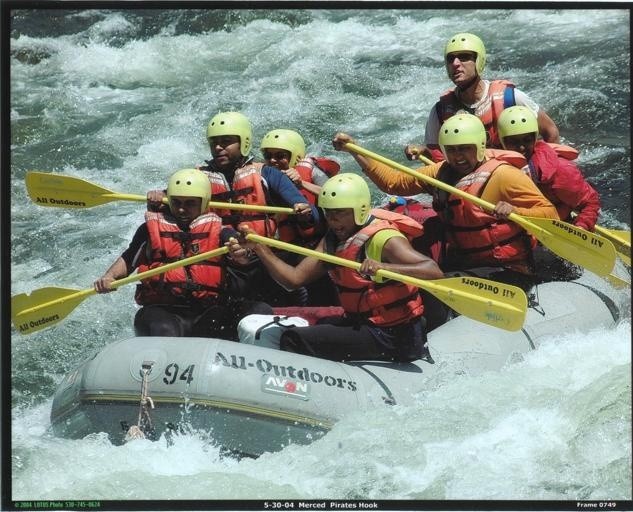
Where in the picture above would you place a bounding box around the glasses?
[447,53,475,64]
[263,151,288,161]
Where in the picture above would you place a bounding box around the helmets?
[167,169,211,216]
[497,105,539,151]
[207,112,254,158]
[439,114,487,163]
[317,173,372,226]
[444,33,486,76]
[259,129,306,169]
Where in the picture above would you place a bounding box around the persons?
[404,106,601,230]
[240,173,442,363]
[259,126,326,207]
[332,113,560,325]
[406,33,559,144]
[95,169,248,335]
[147,113,321,300]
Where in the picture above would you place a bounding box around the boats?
[49,255,631,459]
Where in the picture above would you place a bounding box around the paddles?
[26,172,300,214]
[11,237,242,336]
[237,225,528,332]
[339,142,617,279]
[411,148,633,270]
